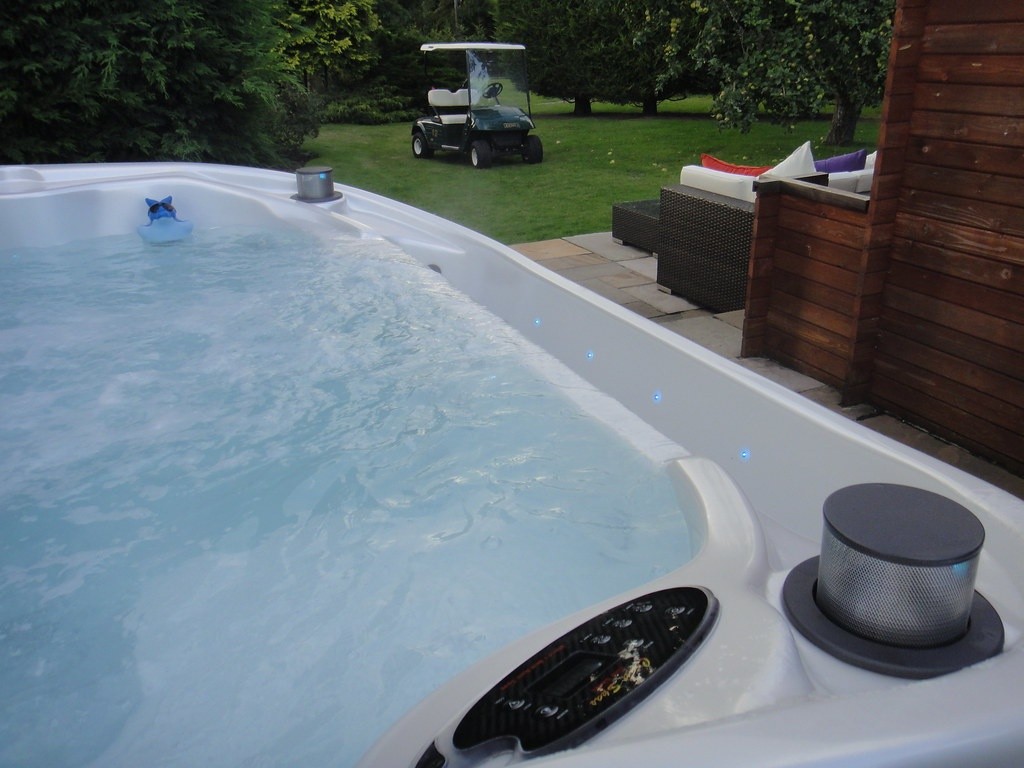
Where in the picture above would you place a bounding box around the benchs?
[428,89,480,124]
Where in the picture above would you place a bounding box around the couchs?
[656,141,877,314]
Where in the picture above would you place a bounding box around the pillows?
[814,148,867,173]
[701,153,772,177]
[757,141,817,177]
